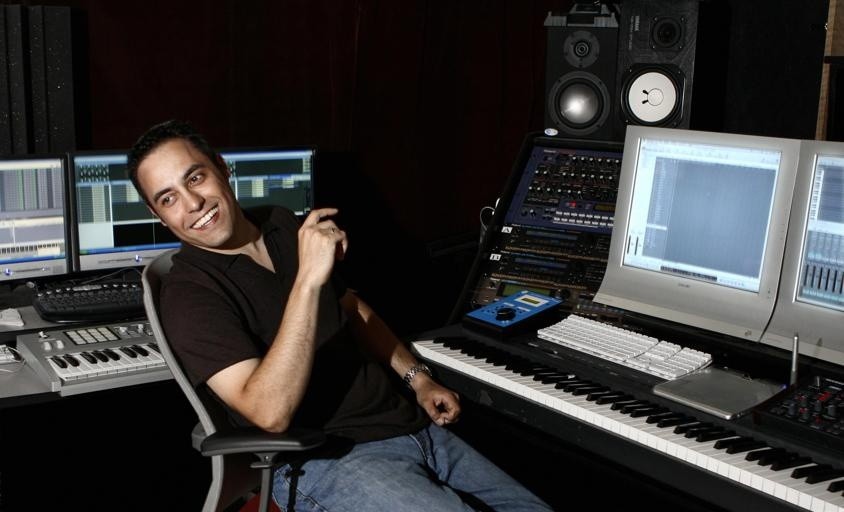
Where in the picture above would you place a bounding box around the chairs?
[142,247,324,511]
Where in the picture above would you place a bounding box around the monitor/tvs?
[758,140,844,366]
[66,148,181,271]
[592,123,802,341]
[216,143,317,218]
[0,154,72,282]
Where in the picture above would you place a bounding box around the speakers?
[611,0,699,141]
[542,0,619,141]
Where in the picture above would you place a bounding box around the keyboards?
[537,314,713,381]
[31,281,146,323]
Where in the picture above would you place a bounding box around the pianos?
[17,321,175,397]
[412,332,840,512]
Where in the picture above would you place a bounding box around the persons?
[127,120,551,512]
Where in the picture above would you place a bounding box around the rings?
[329,226,334,233]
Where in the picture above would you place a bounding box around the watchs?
[405,363,434,385]
